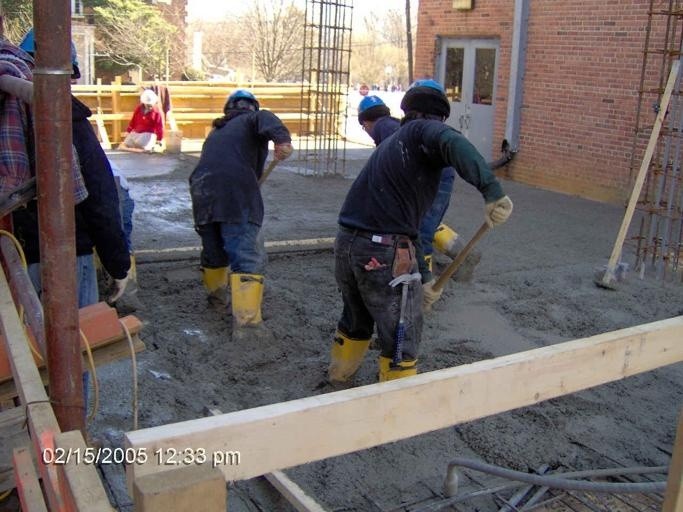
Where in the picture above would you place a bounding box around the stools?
[339,224,375,241]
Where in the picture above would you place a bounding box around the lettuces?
[388,272,422,363]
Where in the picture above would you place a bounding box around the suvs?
[165,130,183,155]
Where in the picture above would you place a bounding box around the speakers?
[199,267,230,293]
[231,273,270,338]
[425,223,481,278]
[327,330,371,382]
[376,356,417,382]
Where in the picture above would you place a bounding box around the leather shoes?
[483,195,512,229]
[422,281,444,308]
[107,268,131,303]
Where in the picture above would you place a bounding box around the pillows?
[140,90,158,105]
[400,78,450,117]
[21,27,81,80]
[224,90,259,110]
[358,96,386,121]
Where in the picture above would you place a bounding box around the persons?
[189,89,294,339]
[324,78,513,382]
[116,89,164,152]
[11,25,130,428]
[358,96,483,284]
